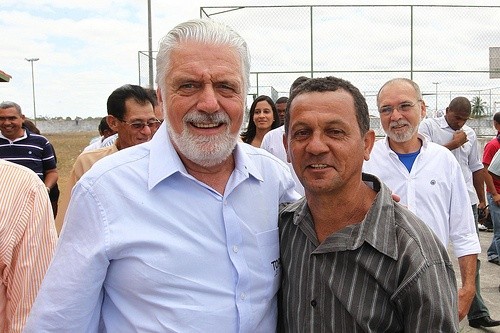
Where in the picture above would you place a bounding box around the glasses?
[378,100,422,115]
[114,114,161,131]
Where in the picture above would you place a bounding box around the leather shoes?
[469,316,500,328]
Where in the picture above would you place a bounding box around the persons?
[3,98,500,328]
[364,79,480,324]
[277,76,462,332]
[0,157,59,333]
[21,19,409,333]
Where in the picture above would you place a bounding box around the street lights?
[24,57,40,127]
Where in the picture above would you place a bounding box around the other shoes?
[490,257,500,266]
[478,224,493,232]
